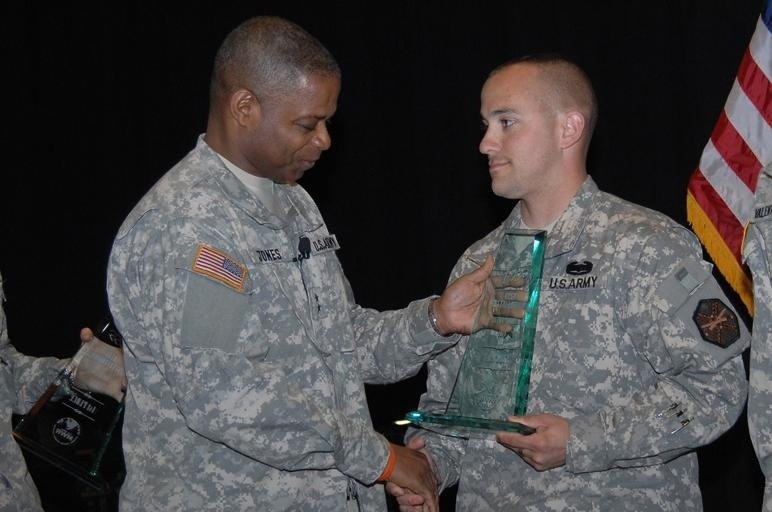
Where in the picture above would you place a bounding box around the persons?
[0,276,129,510]
[748,159,771,511]
[105,14,532,512]
[383,51,750,511]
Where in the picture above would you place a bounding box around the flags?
[686,0,772,319]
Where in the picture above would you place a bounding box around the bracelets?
[429,294,449,338]
[373,441,397,485]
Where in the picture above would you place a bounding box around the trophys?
[7,307,131,499]
[403,227,548,442]
